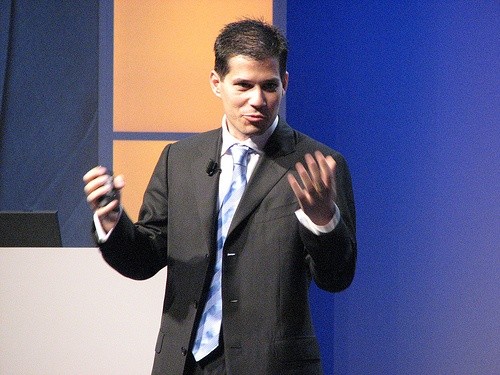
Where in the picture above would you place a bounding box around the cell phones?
[97,165,121,212]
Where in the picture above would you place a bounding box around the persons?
[83,17,359,375]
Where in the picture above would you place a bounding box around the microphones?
[205,158,221,177]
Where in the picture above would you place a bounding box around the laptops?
[0,210,63,247]
